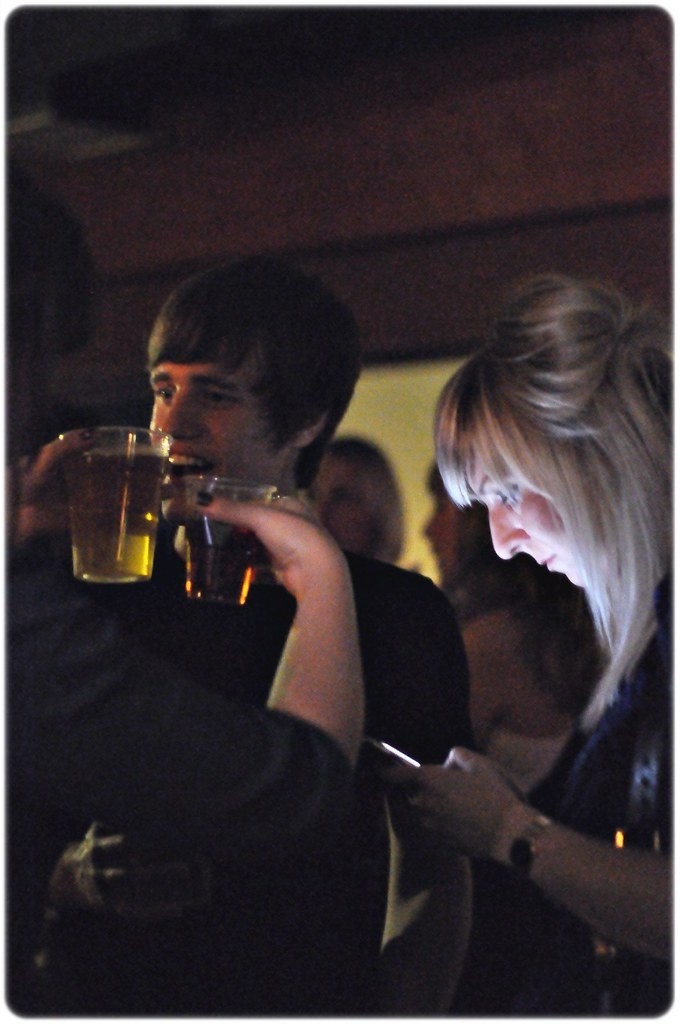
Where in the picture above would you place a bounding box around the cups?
[59,424,173,584]
[187,474,277,605]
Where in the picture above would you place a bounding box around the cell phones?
[361,736,420,769]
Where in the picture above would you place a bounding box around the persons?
[35,253,606,1016]
[383,266,673,1016]
[8,165,365,1016]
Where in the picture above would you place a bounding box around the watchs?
[508,816,554,880]
